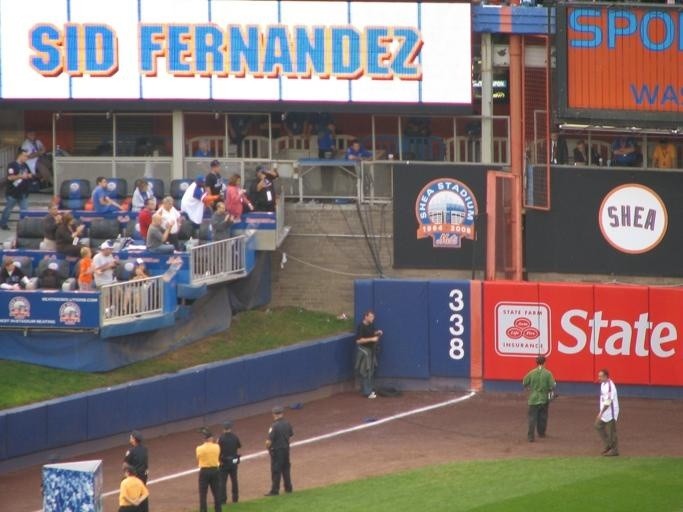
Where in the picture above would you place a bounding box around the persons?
[195,139,215,157]
[218,420,241,505]
[594,368,621,457]
[265,405,295,496]
[225,174,248,219]
[522,355,556,442]
[54,138,157,157]
[230,120,257,158]
[0,172,234,318]
[249,163,279,212]
[122,429,149,485]
[353,309,383,399]
[118,464,150,511]
[343,139,373,194]
[196,431,223,512]
[546,133,679,169]
[205,159,224,210]
[318,117,336,192]
[1,128,53,230]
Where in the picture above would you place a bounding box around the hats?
[210,158,219,165]
[272,406,284,413]
[224,420,232,429]
[197,425,211,438]
[132,430,143,441]
[195,174,207,185]
[99,239,114,250]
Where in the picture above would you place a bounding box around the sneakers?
[600,445,619,456]
[368,391,377,399]
[264,491,279,496]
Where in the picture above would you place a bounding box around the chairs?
[1,176,292,327]
